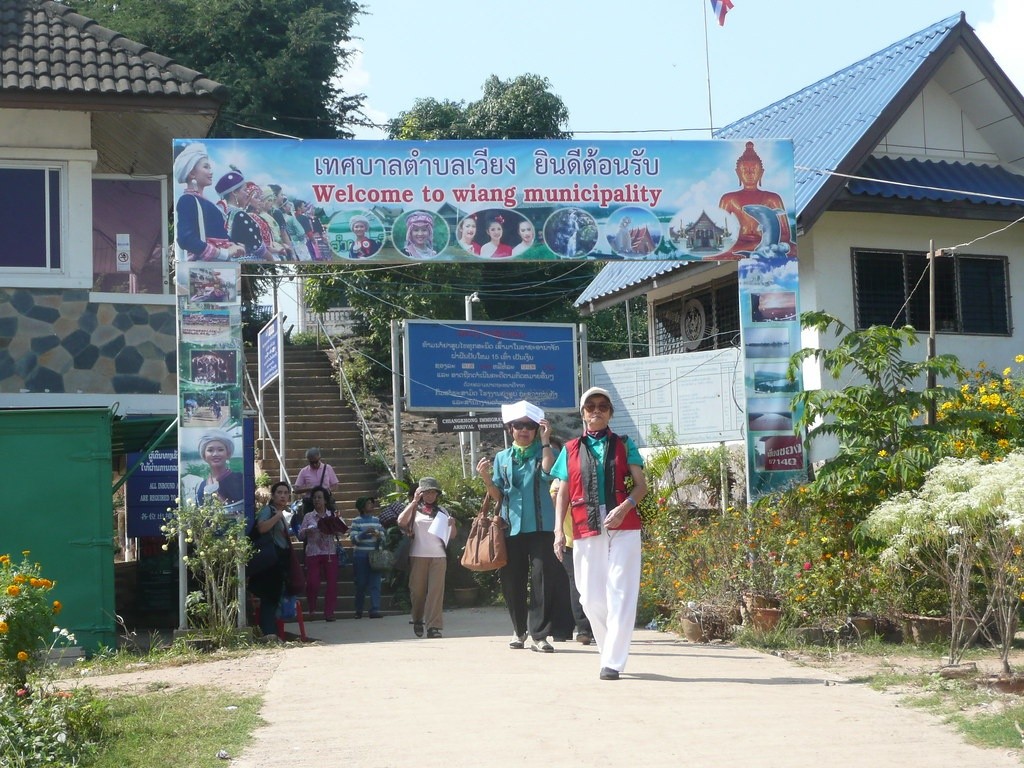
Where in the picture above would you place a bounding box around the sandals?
[509,628,528,650]
[426,627,442,638]
[532,637,554,652]
[414,619,424,637]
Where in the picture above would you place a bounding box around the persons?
[258,183,333,262]
[397,476,457,638]
[540,436,600,646]
[174,142,247,262]
[194,429,245,536]
[511,219,534,256]
[479,211,513,258]
[719,141,799,260]
[401,210,437,258]
[549,387,649,679]
[216,170,276,261]
[475,399,558,653]
[459,212,481,257]
[256,481,290,639]
[348,215,378,259]
[350,496,384,619]
[292,447,340,556]
[244,182,287,262]
[296,485,347,622]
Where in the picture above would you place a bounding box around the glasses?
[309,458,320,465]
[584,403,612,412]
[511,421,538,431]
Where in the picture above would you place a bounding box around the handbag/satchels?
[367,535,389,572]
[334,533,349,567]
[461,486,509,571]
[288,549,304,592]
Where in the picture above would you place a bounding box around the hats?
[356,497,374,514]
[406,212,434,243]
[174,142,312,213]
[580,387,614,419]
[349,216,370,231]
[198,429,234,459]
[418,478,442,495]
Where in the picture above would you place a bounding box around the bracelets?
[627,496,636,507]
[541,443,550,448]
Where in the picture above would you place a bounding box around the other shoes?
[327,614,336,622]
[370,613,383,618]
[576,631,591,645]
[600,666,619,680]
[355,613,361,619]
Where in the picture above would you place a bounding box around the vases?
[851,617,874,638]
[973,676,1024,698]
[680,617,717,643]
[451,587,478,605]
[750,608,784,631]
[896,611,951,645]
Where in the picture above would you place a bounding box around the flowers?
[634,356,1024,676]
